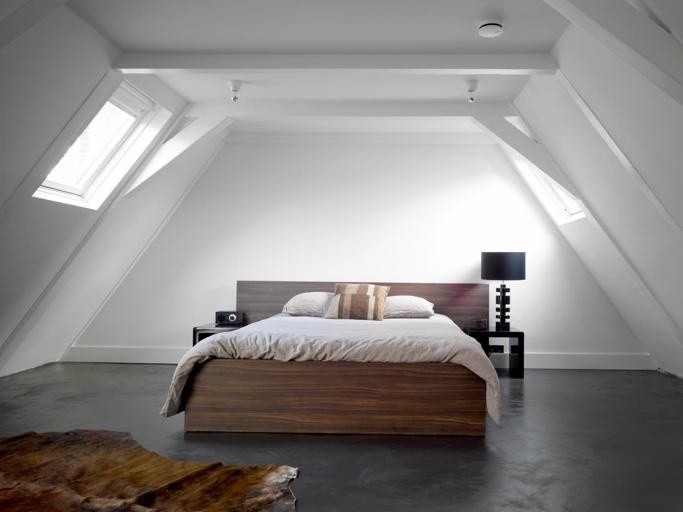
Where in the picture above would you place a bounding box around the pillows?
[281,283,435,322]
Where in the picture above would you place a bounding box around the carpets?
[0,428,301,512]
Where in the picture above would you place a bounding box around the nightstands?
[193,323,239,347]
[464,327,524,379]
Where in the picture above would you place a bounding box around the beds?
[182,280,491,438]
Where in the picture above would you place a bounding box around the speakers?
[214,310,244,326]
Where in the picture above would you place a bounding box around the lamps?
[465,80,477,103]
[481,251,525,332]
[476,20,505,38]
[229,80,241,104]
